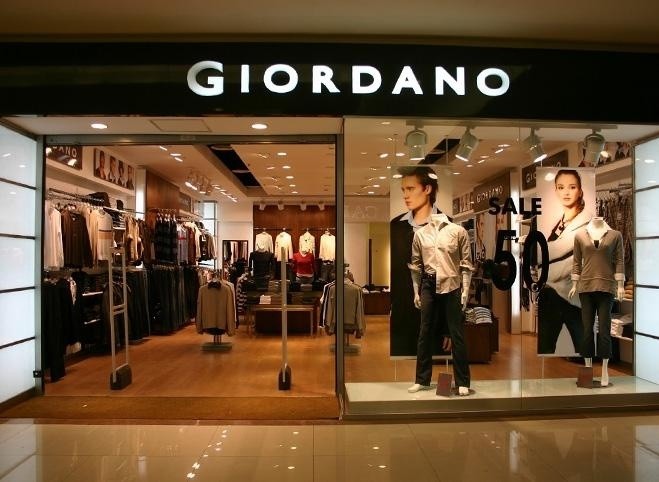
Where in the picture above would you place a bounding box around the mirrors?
[221,239,249,270]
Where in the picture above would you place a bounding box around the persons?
[116,160,125,187]
[105,155,117,183]
[537,170,593,353]
[390,168,452,356]
[567,217,627,386]
[125,166,135,190]
[406,213,472,395]
[95,151,106,180]
[292,247,317,283]
[248,242,276,278]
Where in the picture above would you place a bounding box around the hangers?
[154,206,178,224]
[596,184,634,209]
[149,258,184,273]
[47,189,107,218]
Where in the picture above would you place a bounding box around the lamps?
[184,168,214,197]
[401,118,619,170]
[256,197,328,212]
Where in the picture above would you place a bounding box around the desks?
[247,304,323,338]
